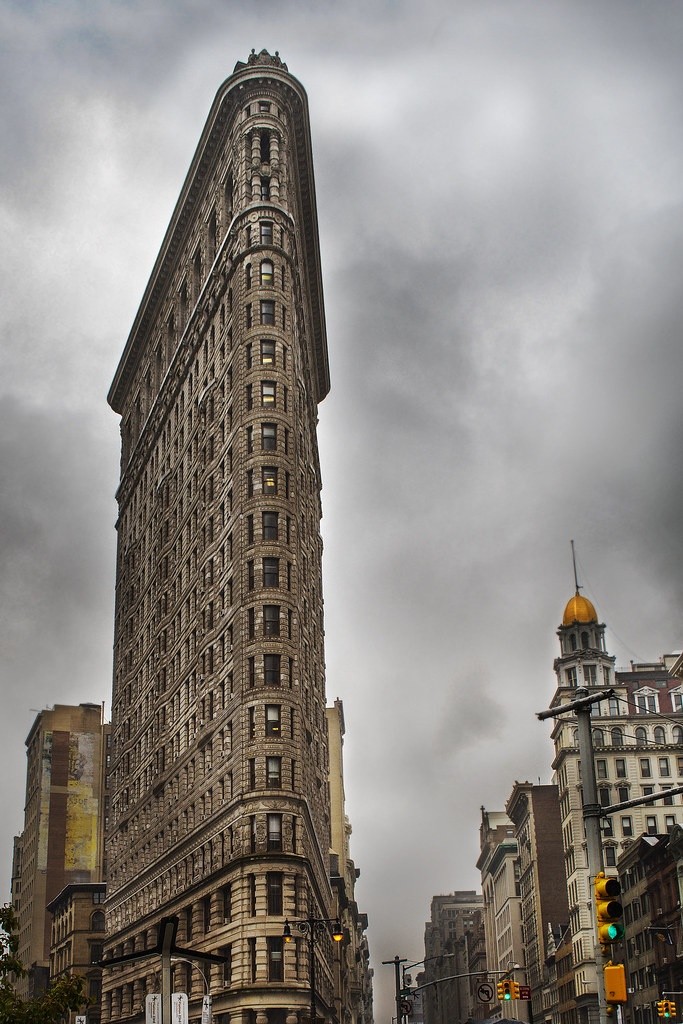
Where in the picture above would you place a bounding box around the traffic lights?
[595,877,625,944]
[497,980,520,1001]
[657,1000,677,1019]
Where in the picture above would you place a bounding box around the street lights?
[170,958,212,1024]
[403,953,455,1024]
[282,904,343,1024]
